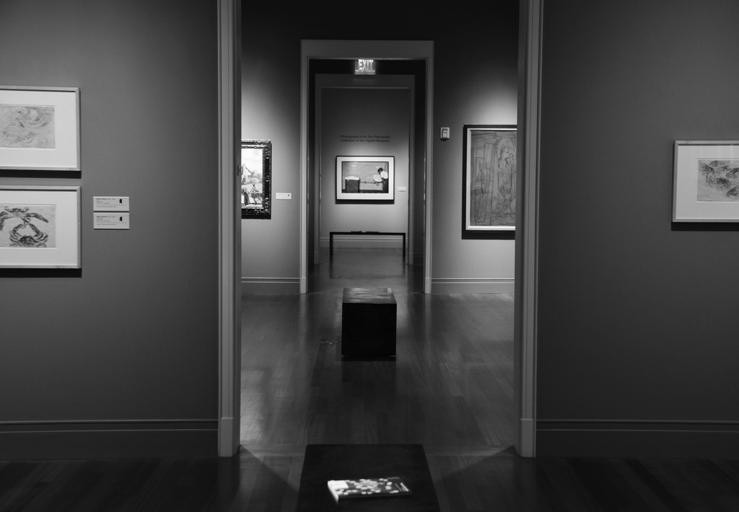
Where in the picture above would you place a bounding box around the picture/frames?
[672,139,739,224]
[240,139,272,219]
[0,84,82,270]
[335,155,395,201]
[462,124,517,240]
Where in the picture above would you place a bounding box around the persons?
[248,184,262,205]
[240,162,250,206]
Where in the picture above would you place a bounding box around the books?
[327,475,414,502]
